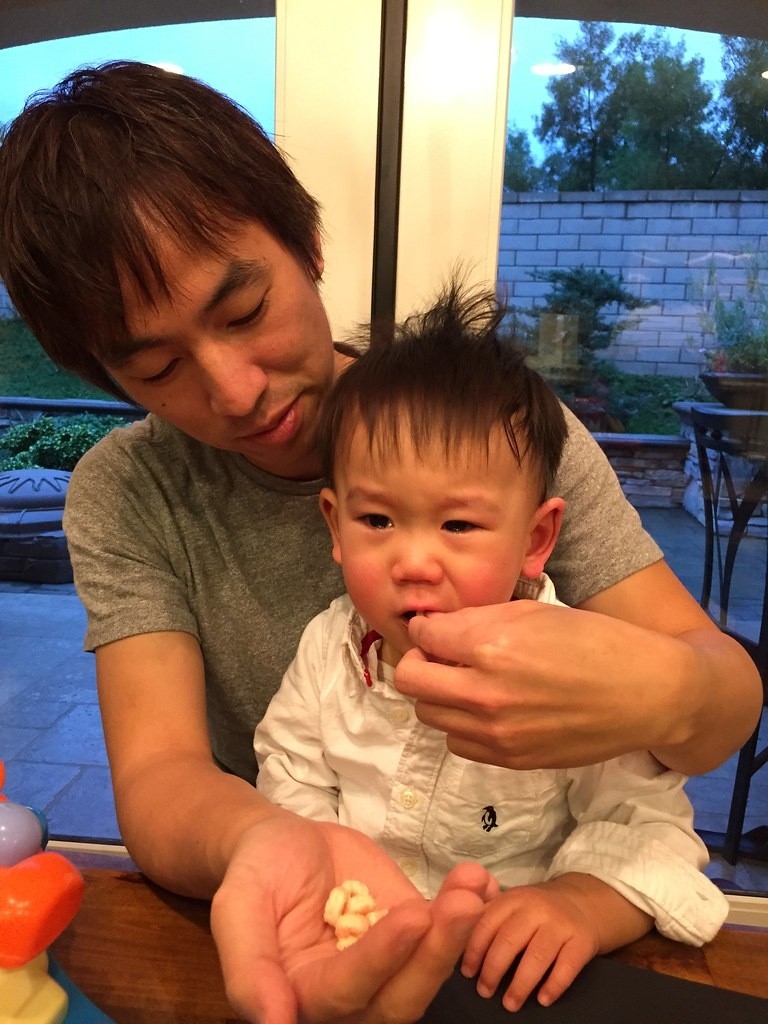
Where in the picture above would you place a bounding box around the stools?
[690,403,768,895]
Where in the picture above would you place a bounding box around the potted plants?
[687,237,768,411]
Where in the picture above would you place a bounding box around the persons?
[249,274,730,1011]
[0,59,763,1024]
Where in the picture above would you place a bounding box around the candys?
[323,879,390,950]
